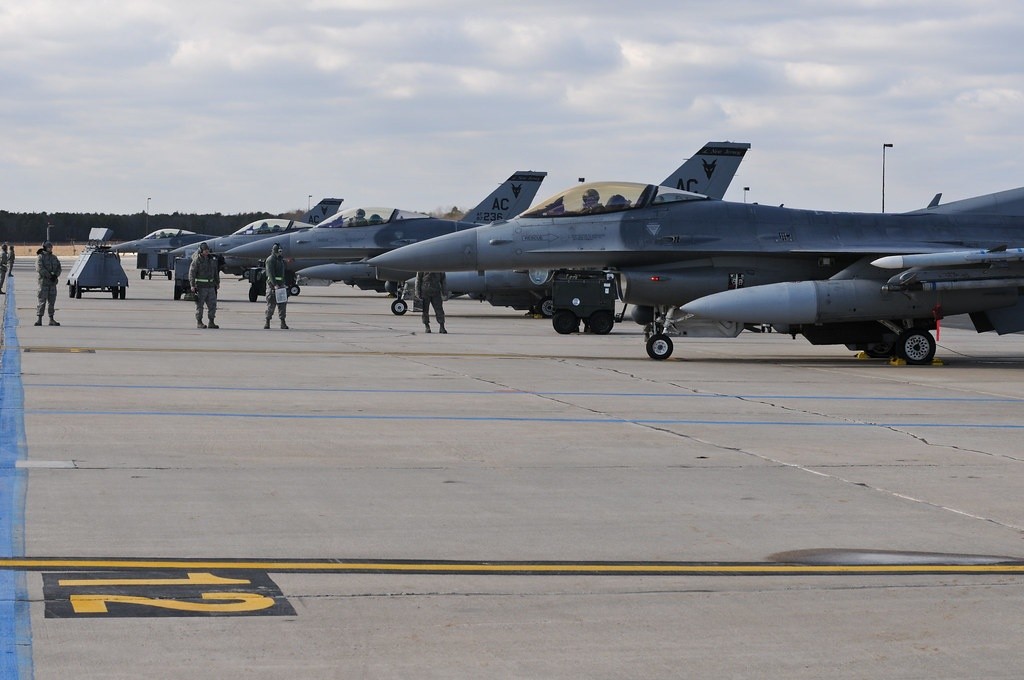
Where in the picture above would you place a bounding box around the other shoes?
[9,273,13,276]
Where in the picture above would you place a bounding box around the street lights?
[881,143,893,213]
[744,186,750,203]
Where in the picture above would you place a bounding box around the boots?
[264,320,270,329]
[440,324,447,333]
[208,319,219,328]
[425,324,431,333]
[197,320,207,328]
[281,321,288,329]
[49,316,60,326]
[0,288,6,294]
[34,316,42,326]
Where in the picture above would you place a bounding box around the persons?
[582,190,605,213]
[350,209,368,227]
[414,272,447,335]
[7,246,15,277]
[188,242,220,328]
[0,245,9,294]
[263,243,290,330]
[33,241,60,326]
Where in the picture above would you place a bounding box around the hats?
[201,243,209,250]
[42,241,52,248]
[272,243,283,253]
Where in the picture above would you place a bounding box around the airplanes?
[365,140,1024,366]
[99,169,660,336]
